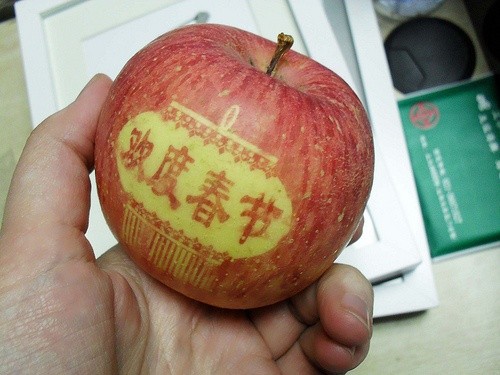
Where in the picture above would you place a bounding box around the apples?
[95,22,376,308]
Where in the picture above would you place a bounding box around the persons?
[0,73,374,375]
